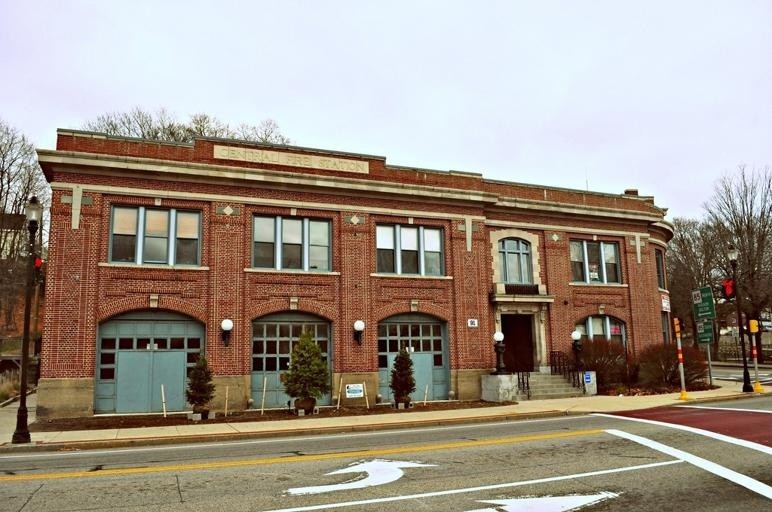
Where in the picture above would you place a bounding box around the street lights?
[11,192,44,444]
[728,243,755,392]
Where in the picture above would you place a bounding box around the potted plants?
[280,325,334,415]
[389,344,417,409]
[185,354,217,420]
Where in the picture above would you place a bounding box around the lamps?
[354,320,365,346]
[222,319,233,346]
